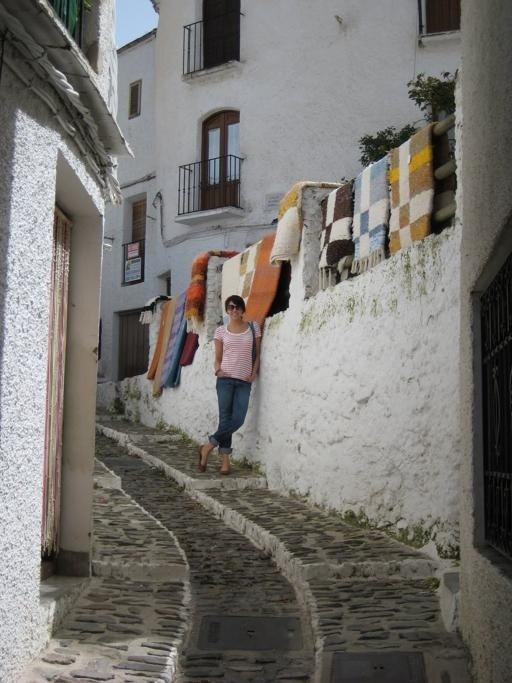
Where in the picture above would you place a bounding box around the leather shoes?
[220,471,229,475]
[199,446,206,472]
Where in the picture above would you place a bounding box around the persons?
[196,294,261,476]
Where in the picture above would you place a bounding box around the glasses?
[228,305,242,310]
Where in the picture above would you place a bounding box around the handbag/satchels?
[248,321,256,362]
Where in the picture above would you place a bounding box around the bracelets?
[215,369,222,376]
